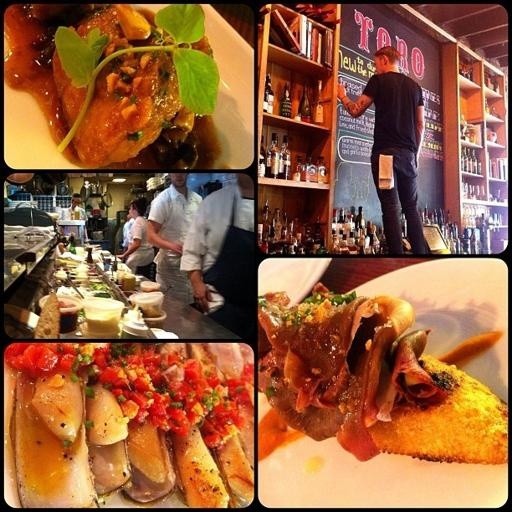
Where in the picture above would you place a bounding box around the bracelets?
[341,95,347,101]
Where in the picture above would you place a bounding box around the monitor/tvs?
[423,224,450,251]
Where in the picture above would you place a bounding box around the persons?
[338,45,432,258]
[114,172,257,339]
[57,193,88,240]
[475,217,485,254]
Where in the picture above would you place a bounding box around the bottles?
[71,211,81,219]
[333,206,509,254]
[263,76,327,256]
[455,63,506,203]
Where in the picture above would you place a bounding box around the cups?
[45,235,166,336]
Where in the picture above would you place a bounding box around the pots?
[11,172,113,208]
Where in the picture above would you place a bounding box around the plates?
[257,255,508,512]
[4,2,253,170]
[259,257,332,323]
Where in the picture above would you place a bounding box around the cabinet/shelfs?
[258,4,508,255]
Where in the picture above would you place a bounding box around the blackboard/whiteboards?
[334,4,445,235]
